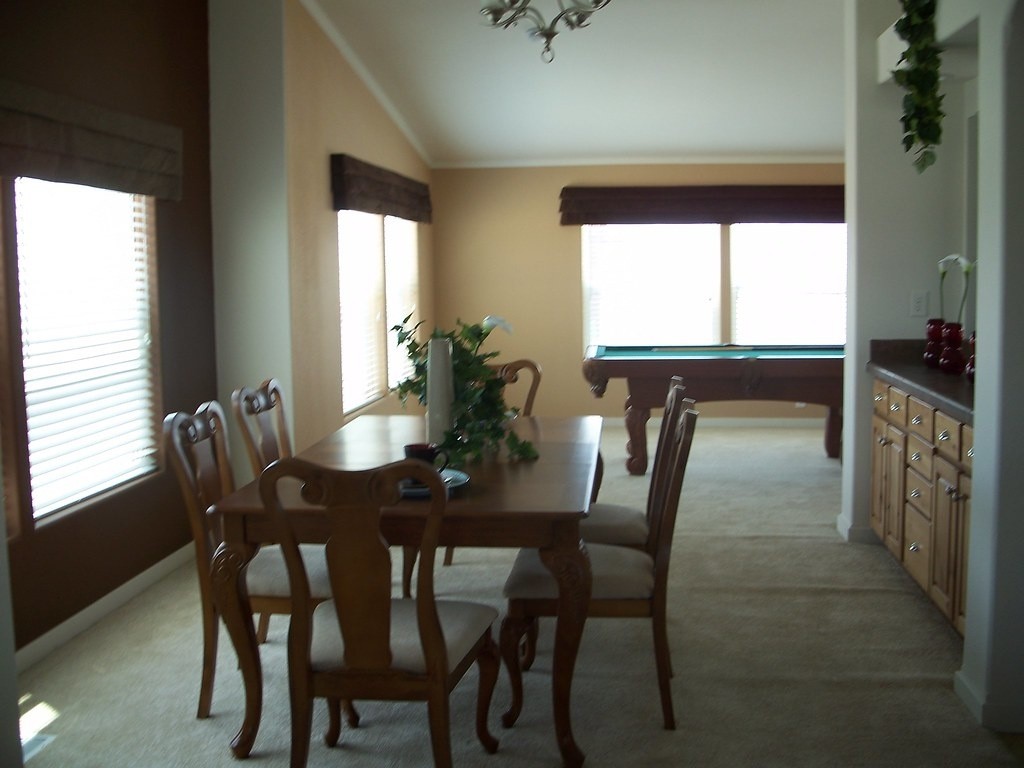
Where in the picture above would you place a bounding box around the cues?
[651,345,845,351]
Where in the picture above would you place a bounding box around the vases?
[453,436,486,490]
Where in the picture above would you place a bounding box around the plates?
[403,468,472,497]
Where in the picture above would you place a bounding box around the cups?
[404,443,451,489]
[425,338,458,445]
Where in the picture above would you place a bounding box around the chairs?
[499,399,697,732]
[162,402,361,730]
[254,455,499,768]
[443,358,541,566]
[520,375,685,656]
[233,377,295,642]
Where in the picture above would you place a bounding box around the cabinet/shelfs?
[865,381,977,636]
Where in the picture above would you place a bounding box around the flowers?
[386,297,539,465]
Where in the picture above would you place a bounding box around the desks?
[585,343,846,474]
[206,414,602,768]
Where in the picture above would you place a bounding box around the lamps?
[478,0,607,63]
